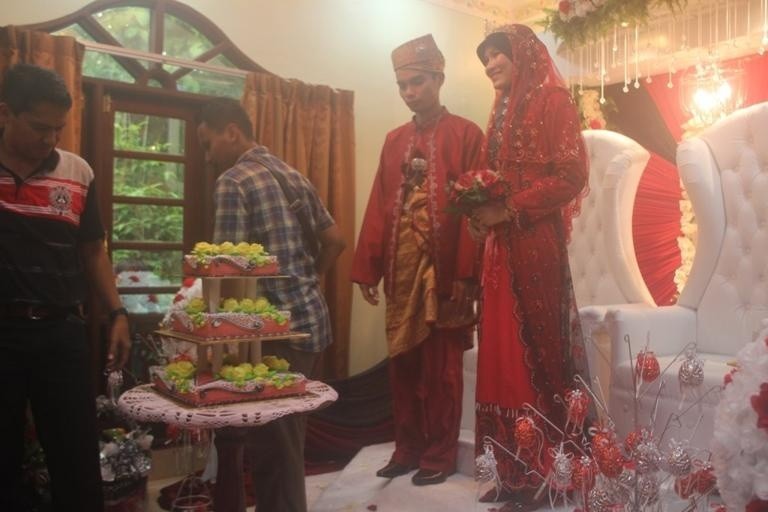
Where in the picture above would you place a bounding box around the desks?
[115,379,339,512]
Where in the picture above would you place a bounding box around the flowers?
[535,0,688,53]
[446,169,510,236]
[577,89,606,133]
[401,146,430,188]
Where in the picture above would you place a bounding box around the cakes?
[152,243,308,407]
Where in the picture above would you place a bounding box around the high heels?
[479,486,544,512]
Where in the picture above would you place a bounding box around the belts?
[10,305,50,321]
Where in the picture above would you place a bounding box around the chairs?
[455,129,656,481]
[605,100,768,512]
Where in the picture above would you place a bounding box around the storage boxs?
[101,463,151,511]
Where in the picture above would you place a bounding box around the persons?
[454,19,607,512]
[346,33,490,488]
[0,61,135,511]
[190,95,349,512]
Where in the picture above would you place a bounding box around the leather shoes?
[376,462,446,485]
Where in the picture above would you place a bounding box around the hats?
[392,33,445,73]
[476,32,513,67]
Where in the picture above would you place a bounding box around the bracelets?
[110,307,129,319]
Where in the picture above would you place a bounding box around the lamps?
[677,49,746,136]
